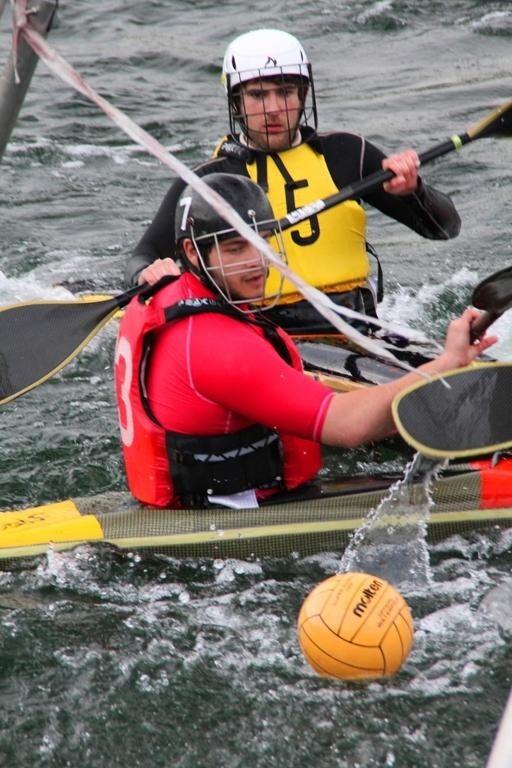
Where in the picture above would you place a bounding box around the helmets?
[174,174,287,315]
[222,28,318,152]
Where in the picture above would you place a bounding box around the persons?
[120,26,466,385]
[112,171,501,513]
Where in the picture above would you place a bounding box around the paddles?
[469,267,512,347]
[388,362,512,464]
[0,92,512,404]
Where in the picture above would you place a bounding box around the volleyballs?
[295,571,417,684]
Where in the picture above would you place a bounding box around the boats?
[0,454,512,573]
